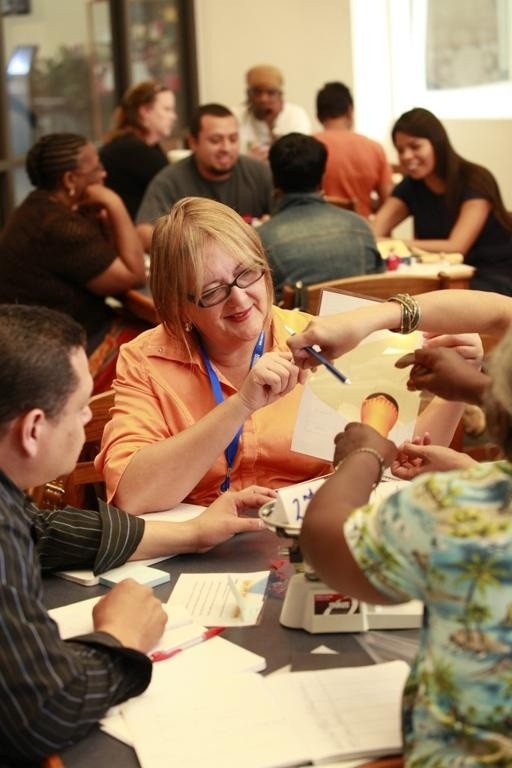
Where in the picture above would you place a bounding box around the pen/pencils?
[151,625,225,663]
[286,327,350,386]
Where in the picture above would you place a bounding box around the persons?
[91,196,511,513]
[0,61,512,398]
[285,282,511,768]
[1,303,166,768]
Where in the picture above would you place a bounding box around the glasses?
[185,262,265,307]
